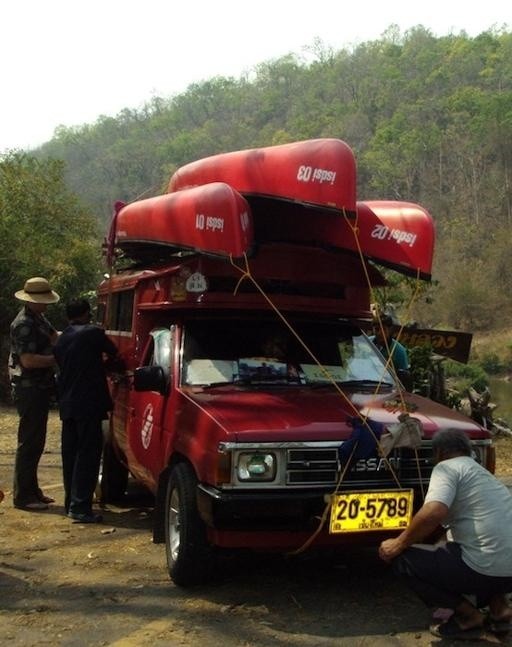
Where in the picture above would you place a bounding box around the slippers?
[428,617,483,640]
[485,612,512,632]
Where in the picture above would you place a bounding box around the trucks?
[98,243,498,589]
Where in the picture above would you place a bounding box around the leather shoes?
[68,510,104,522]
[15,500,49,509]
[40,495,56,502]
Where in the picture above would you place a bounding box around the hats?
[14,276,60,303]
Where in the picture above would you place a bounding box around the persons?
[51,298,118,524]
[8,276,60,511]
[379,426,512,637]
[369,313,412,393]
